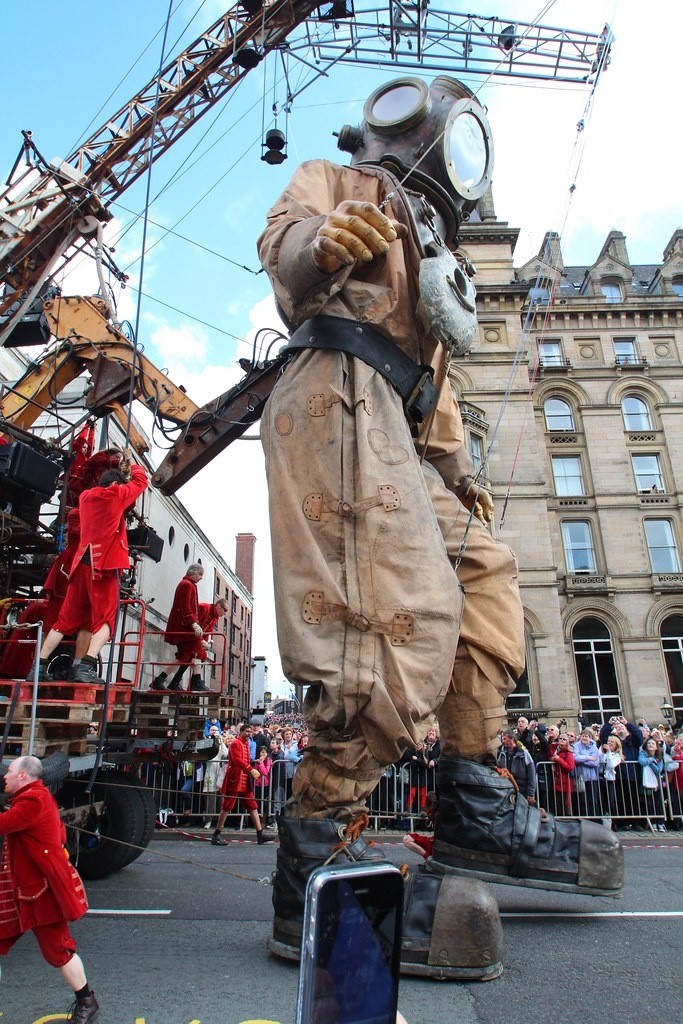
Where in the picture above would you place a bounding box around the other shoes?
[363,822,386,830]
[257,832,275,844]
[184,810,191,815]
[201,821,277,830]
[652,823,667,832]
[420,810,426,816]
[211,835,228,846]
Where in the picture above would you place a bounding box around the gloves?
[124,449,133,462]
[201,639,209,650]
[120,460,131,471]
[192,622,203,638]
[250,769,261,779]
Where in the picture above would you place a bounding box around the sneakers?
[66,989,98,1024]
[67,665,80,681]
[191,681,209,691]
[27,659,53,681]
[149,677,166,690]
[166,679,183,691]
[73,664,105,684]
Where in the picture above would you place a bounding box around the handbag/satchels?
[642,758,659,791]
[573,775,585,792]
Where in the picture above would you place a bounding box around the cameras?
[614,717,620,720]
[561,719,566,725]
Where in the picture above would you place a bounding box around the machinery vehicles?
[1,0,612,880]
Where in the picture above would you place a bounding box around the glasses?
[221,605,228,612]
[559,738,567,740]
[567,734,574,737]
[547,728,554,731]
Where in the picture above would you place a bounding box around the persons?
[256,76,626,980]
[497,716,683,832]
[0,417,148,1024]
[178,714,308,845]
[148,564,229,690]
[366,722,434,861]
